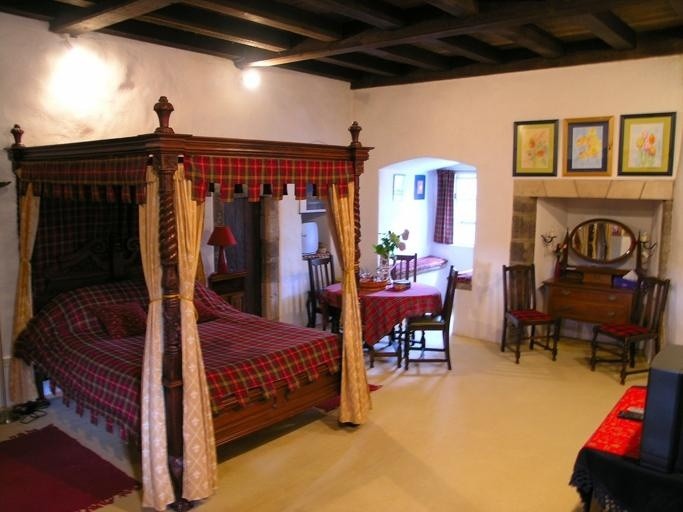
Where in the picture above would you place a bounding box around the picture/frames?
[413,174,426,200]
[561,115,616,177]
[511,118,560,177]
[392,172,410,203]
[617,110,677,177]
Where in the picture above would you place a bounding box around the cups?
[361,272,373,282]
[374,269,385,283]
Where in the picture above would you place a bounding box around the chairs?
[386,253,420,286]
[304,254,343,331]
[590,276,670,388]
[500,261,563,364]
[405,264,459,371]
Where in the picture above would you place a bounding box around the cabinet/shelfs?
[569,343,683,512]
[542,270,645,368]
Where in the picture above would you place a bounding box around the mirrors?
[567,217,637,262]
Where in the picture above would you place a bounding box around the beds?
[9,97,376,512]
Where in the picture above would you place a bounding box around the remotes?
[628,406,645,413]
[618,409,644,421]
